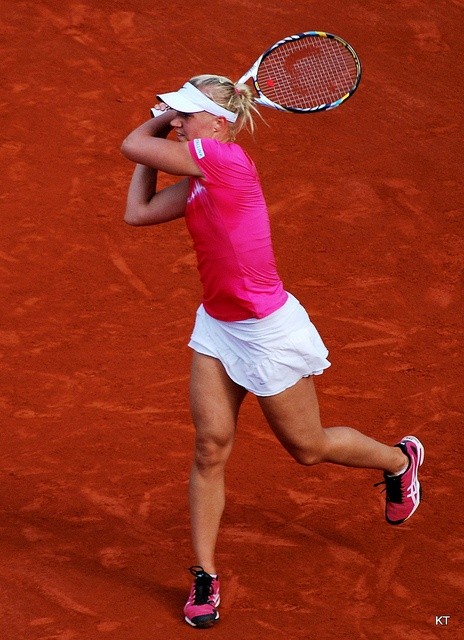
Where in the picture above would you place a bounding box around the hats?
[156,81,239,124]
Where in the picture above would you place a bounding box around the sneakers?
[183,565,221,628]
[373,435,425,525]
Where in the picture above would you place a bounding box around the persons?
[120,74,425,629]
